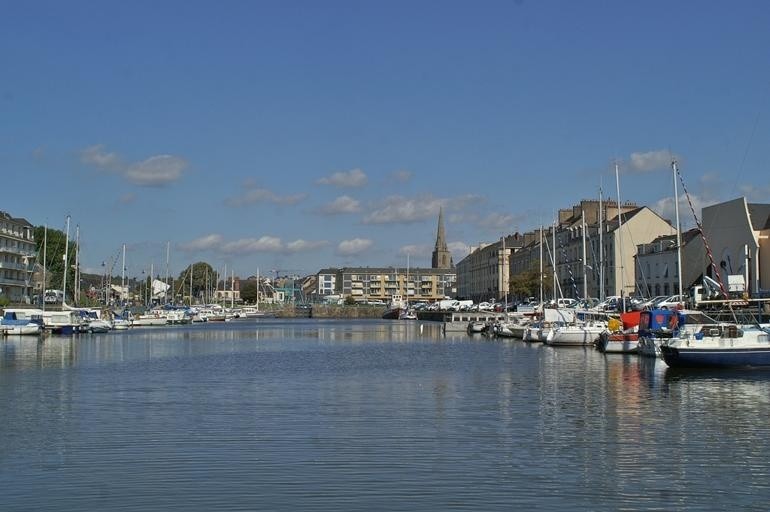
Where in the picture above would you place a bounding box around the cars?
[415,298,519,311]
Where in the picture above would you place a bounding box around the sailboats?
[1,215,265,334]
[384,253,418,321]
